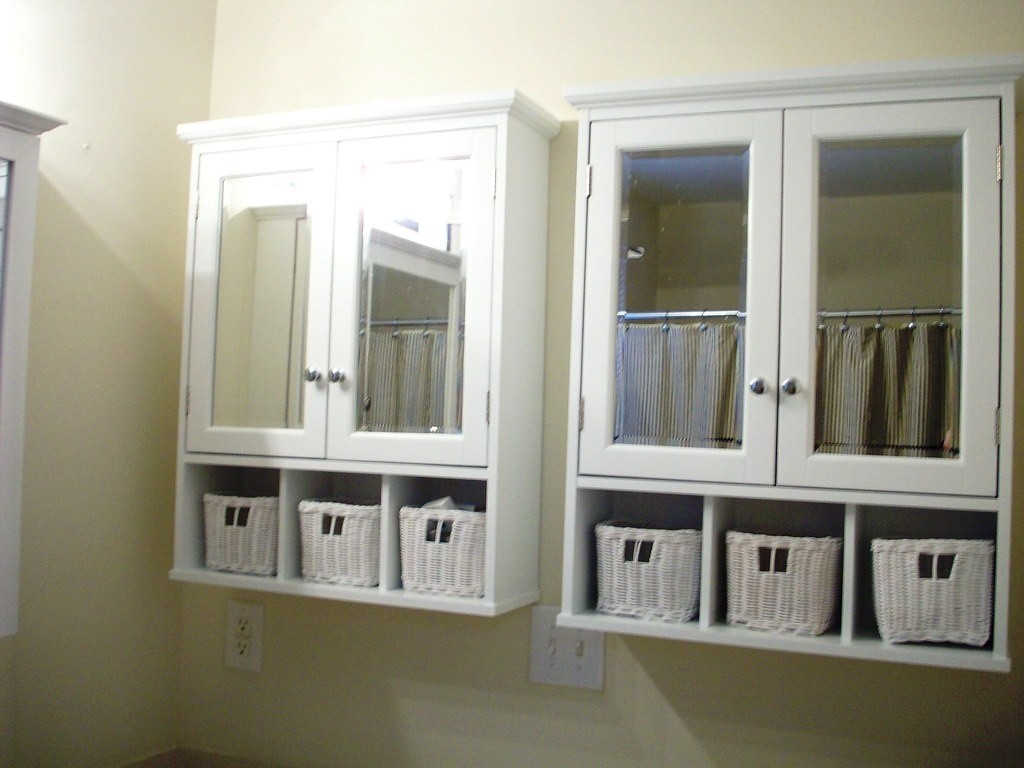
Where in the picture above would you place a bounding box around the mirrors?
[210,168,316,432]
[611,140,750,452]
[352,155,472,436]
[812,136,960,461]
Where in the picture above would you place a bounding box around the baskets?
[722,527,842,635]
[597,517,703,622]
[298,498,381,586]
[399,503,486,598]
[203,488,279,577]
[870,537,994,646]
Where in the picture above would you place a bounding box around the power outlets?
[222,599,265,674]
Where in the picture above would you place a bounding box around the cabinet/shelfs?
[556,56,1024,676]
[168,91,561,616]
